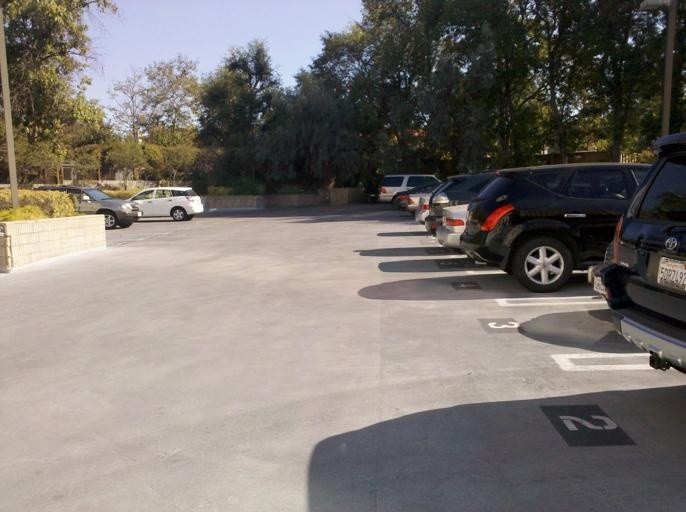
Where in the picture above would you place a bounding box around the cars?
[124,185,205,221]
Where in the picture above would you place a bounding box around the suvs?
[36,185,142,230]
